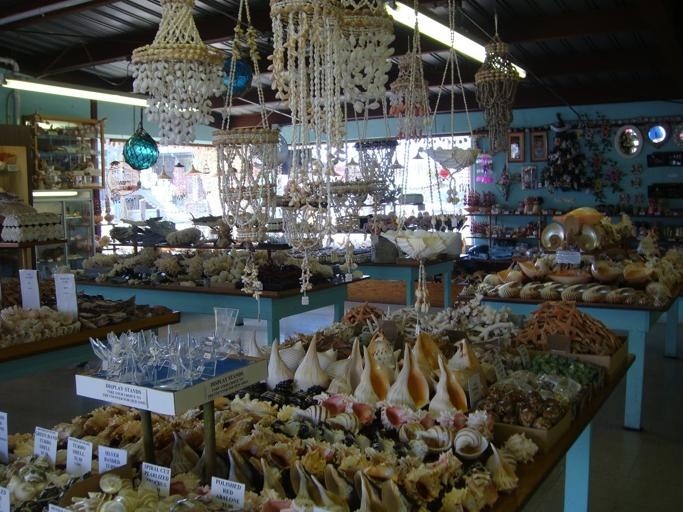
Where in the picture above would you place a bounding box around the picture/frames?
[529,131,548,162]
[506,133,525,162]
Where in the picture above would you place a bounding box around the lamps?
[123,107,159,170]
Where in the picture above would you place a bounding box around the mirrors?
[612,125,643,160]
[644,124,670,148]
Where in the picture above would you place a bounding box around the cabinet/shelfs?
[0,102,106,283]
